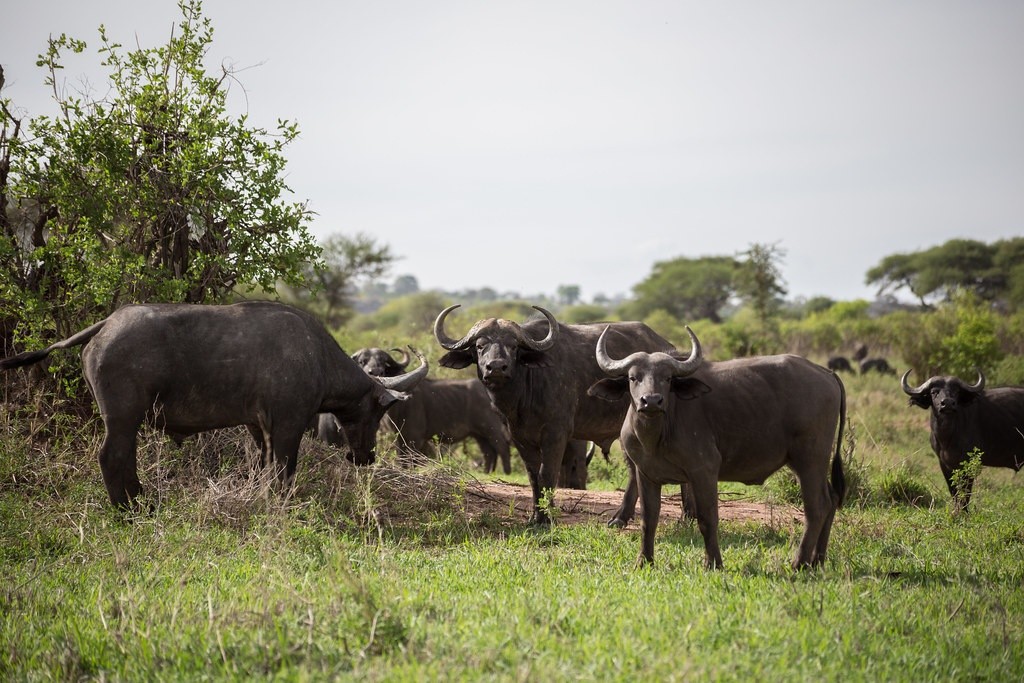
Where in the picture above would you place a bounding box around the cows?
[901,367,1024,512]
[433,304,678,525]
[308,346,513,475]
[595,324,847,573]
[827,341,897,377]
[0,300,428,525]
